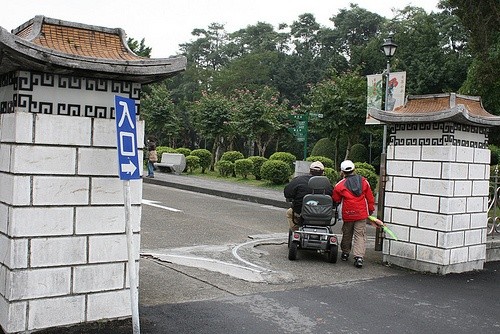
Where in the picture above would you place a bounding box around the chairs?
[301,175,333,225]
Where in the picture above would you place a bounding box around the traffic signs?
[289,115,308,143]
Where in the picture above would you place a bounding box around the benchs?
[153,152,186,175]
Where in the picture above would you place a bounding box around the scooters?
[286,176,340,263]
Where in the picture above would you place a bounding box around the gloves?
[369,211,373,215]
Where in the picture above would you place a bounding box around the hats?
[341,160,354,171]
[310,161,324,170]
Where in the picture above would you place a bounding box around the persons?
[145,138,156,178]
[284,161,333,231]
[332,160,375,265]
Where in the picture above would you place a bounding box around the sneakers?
[341,252,350,261]
[354,256,363,267]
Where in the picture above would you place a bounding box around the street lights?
[374,34,399,251]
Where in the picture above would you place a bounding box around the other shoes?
[146,175,154,178]
